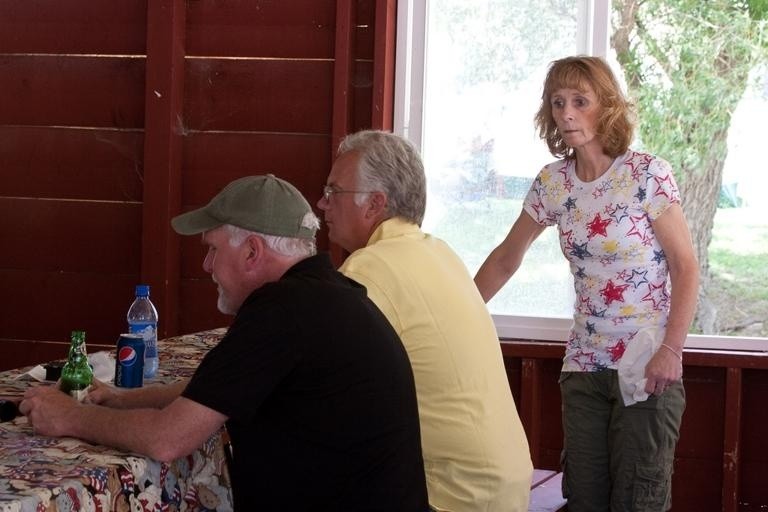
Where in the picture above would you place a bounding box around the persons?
[315,130,533,512]
[472,54,700,512]
[18,175,432,512]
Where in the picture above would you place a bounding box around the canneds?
[115,334,146,387]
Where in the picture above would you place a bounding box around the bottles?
[126,284,159,379]
[59,329,96,404]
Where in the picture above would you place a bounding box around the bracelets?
[663,344,685,361]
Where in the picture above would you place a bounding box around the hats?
[171,174,317,239]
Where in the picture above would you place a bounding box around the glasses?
[324,187,377,199]
